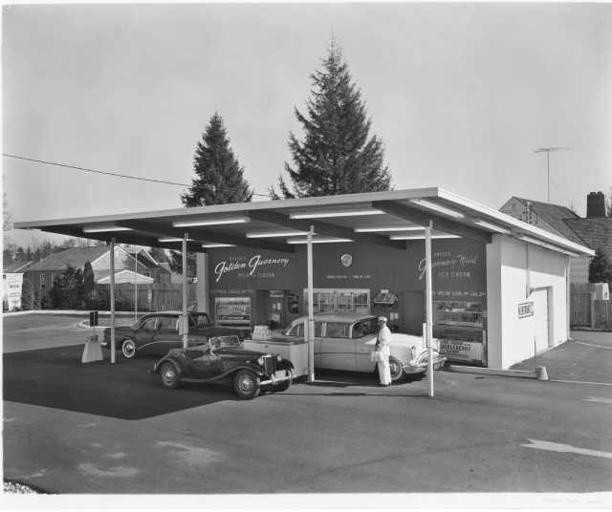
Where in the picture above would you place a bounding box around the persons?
[370,316,392,386]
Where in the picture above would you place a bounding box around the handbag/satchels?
[370,350,384,363]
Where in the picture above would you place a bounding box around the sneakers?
[376,381,391,387]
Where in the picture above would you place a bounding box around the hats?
[378,315,387,323]
